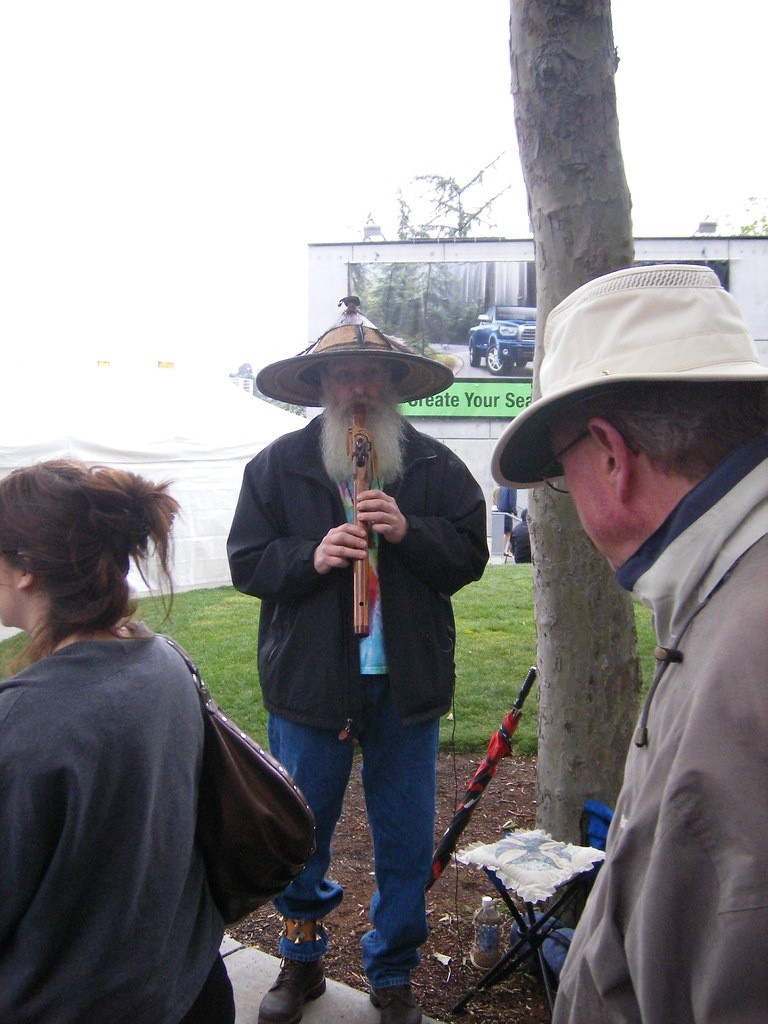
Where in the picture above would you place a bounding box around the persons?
[491,264,768,1024]
[497,484,531,563]
[0,458,236,1024]
[226,313,489,1024]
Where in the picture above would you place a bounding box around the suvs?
[470,305,537,376]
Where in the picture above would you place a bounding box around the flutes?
[351,406,369,638]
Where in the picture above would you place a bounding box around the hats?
[256,291,456,409]
[490,264,768,489]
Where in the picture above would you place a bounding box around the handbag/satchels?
[155,633,316,928]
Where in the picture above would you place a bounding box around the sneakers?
[257,957,326,1024]
[370,981,421,1024]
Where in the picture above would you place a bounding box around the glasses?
[533,425,640,494]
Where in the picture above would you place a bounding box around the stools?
[450,827,608,1021]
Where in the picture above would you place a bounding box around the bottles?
[472,896,500,968]
